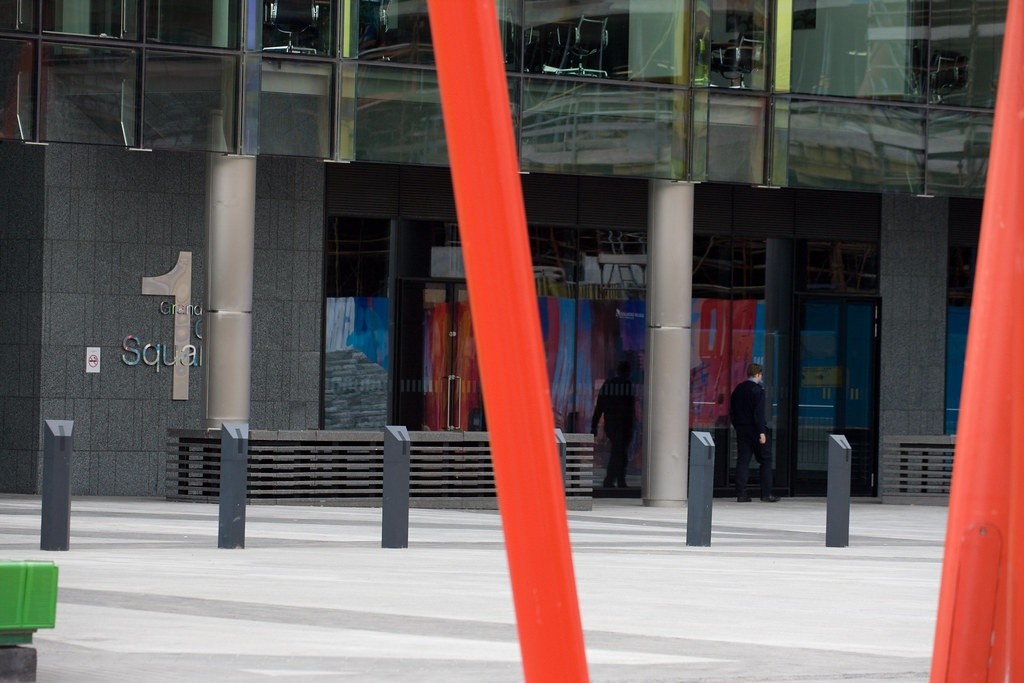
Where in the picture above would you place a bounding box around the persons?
[730,362,780,502]
[588,362,638,489]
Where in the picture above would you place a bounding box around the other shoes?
[760,494,781,502]
[737,496,752,502]
